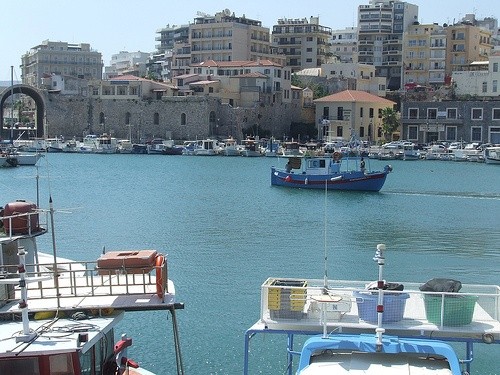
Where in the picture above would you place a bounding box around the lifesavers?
[286,176,290,182]
[155,256,166,299]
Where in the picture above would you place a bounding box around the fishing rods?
[15,275,115,357]
[324,180,329,297]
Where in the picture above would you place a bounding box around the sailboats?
[1,66,45,164]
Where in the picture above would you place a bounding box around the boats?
[270,134,390,192]
[0,152,18,168]
[9,140,500,162]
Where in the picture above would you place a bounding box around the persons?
[285,162,292,173]
[360,157,365,174]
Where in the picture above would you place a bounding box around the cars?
[351,139,500,149]
